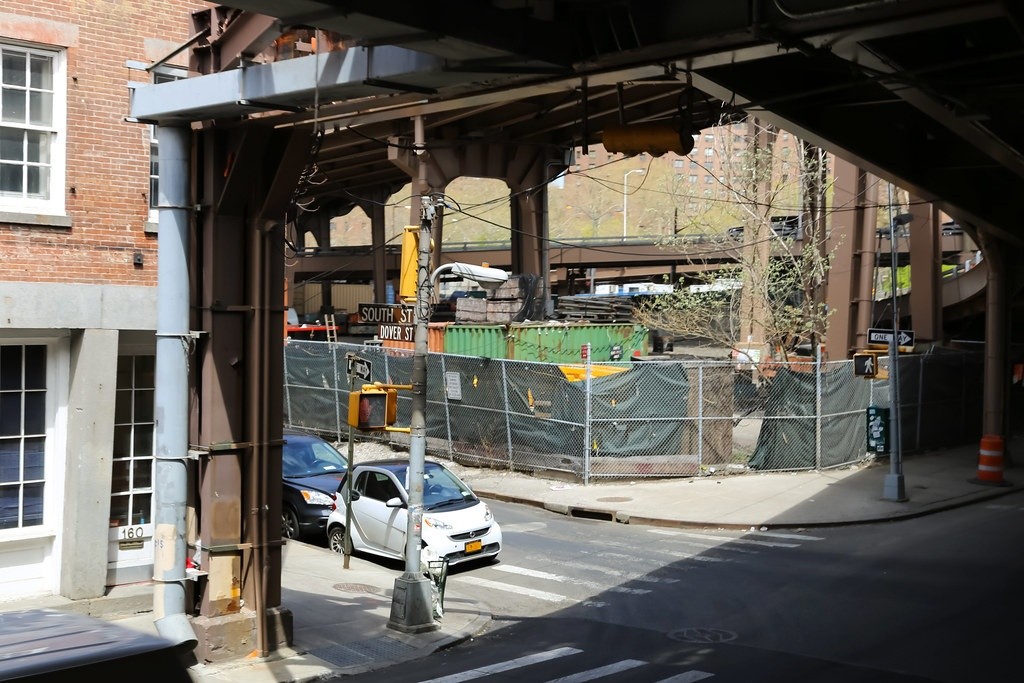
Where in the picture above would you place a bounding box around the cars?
[280,427,349,542]
[326,459,502,577]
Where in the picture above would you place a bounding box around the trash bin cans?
[420,555,450,618]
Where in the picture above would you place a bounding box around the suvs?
[725,215,798,241]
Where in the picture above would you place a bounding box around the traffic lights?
[853,352,878,376]
[347,387,398,429]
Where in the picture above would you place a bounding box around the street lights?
[670,206,708,286]
[391,205,411,245]
[623,169,644,241]
[387,261,509,631]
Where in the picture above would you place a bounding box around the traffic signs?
[867,328,915,347]
[346,355,373,383]
